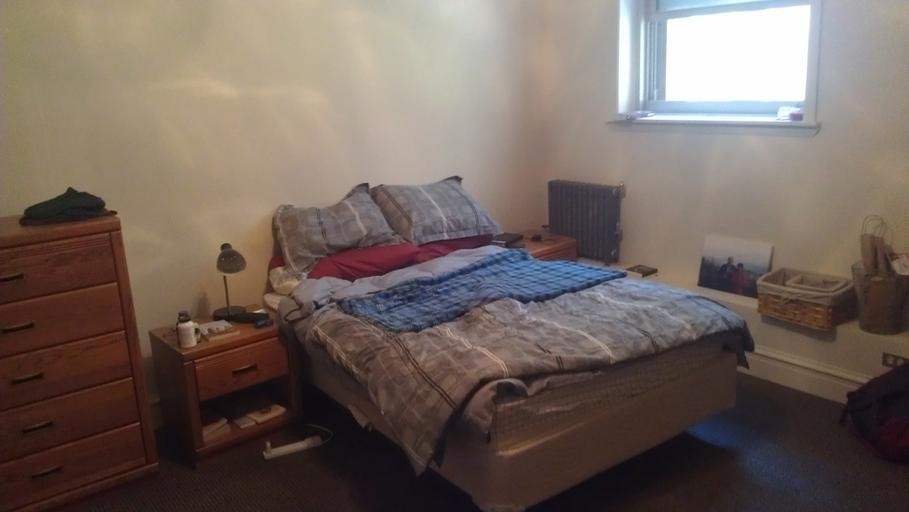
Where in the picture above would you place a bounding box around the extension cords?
[262,435,323,461]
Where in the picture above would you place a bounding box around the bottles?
[177,310,197,350]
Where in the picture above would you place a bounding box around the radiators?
[547,180,625,264]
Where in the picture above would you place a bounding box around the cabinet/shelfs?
[0,222,159,512]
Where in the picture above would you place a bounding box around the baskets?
[756,266,853,332]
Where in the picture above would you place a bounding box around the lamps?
[211,242,246,322]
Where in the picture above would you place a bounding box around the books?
[622,262,654,279]
[200,395,287,444]
[197,316,240,342]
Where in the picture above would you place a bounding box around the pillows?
[269,174,504,293]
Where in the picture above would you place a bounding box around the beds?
[262,174,753,512]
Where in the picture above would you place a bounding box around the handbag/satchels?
[860,213,888,278]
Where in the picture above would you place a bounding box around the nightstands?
[518,230,577,261]
[149,304,303,469]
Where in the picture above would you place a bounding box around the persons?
[717,253,748,294]
